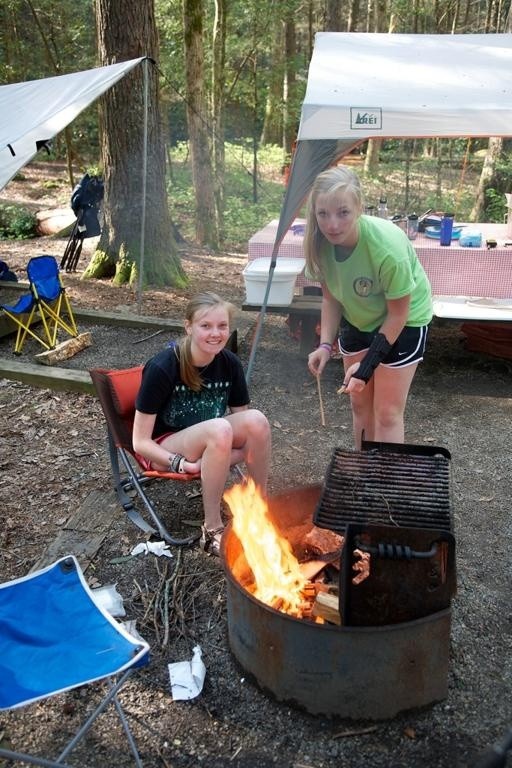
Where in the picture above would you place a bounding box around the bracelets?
[171,452,182,470]
[320,344,332,355]
[318,340,334,347]
[179,457,187,476]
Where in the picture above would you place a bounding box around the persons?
[132,291,272,557]
[303,166,433,451]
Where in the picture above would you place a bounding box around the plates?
[425,226,462,240]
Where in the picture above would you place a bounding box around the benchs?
[241,295,512,359]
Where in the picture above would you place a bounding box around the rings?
[342,382,348,387]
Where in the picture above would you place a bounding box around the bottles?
[439,212,454,246]
[364,196,418,240]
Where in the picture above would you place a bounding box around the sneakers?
[200,523,225,558]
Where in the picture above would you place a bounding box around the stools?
[0,555,151,768]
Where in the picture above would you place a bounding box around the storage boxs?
[242,257,306,308]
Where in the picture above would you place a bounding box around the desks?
[249,217,512,297]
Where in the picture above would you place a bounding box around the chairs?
[89,368,247,545]
[0,255,78,356]
